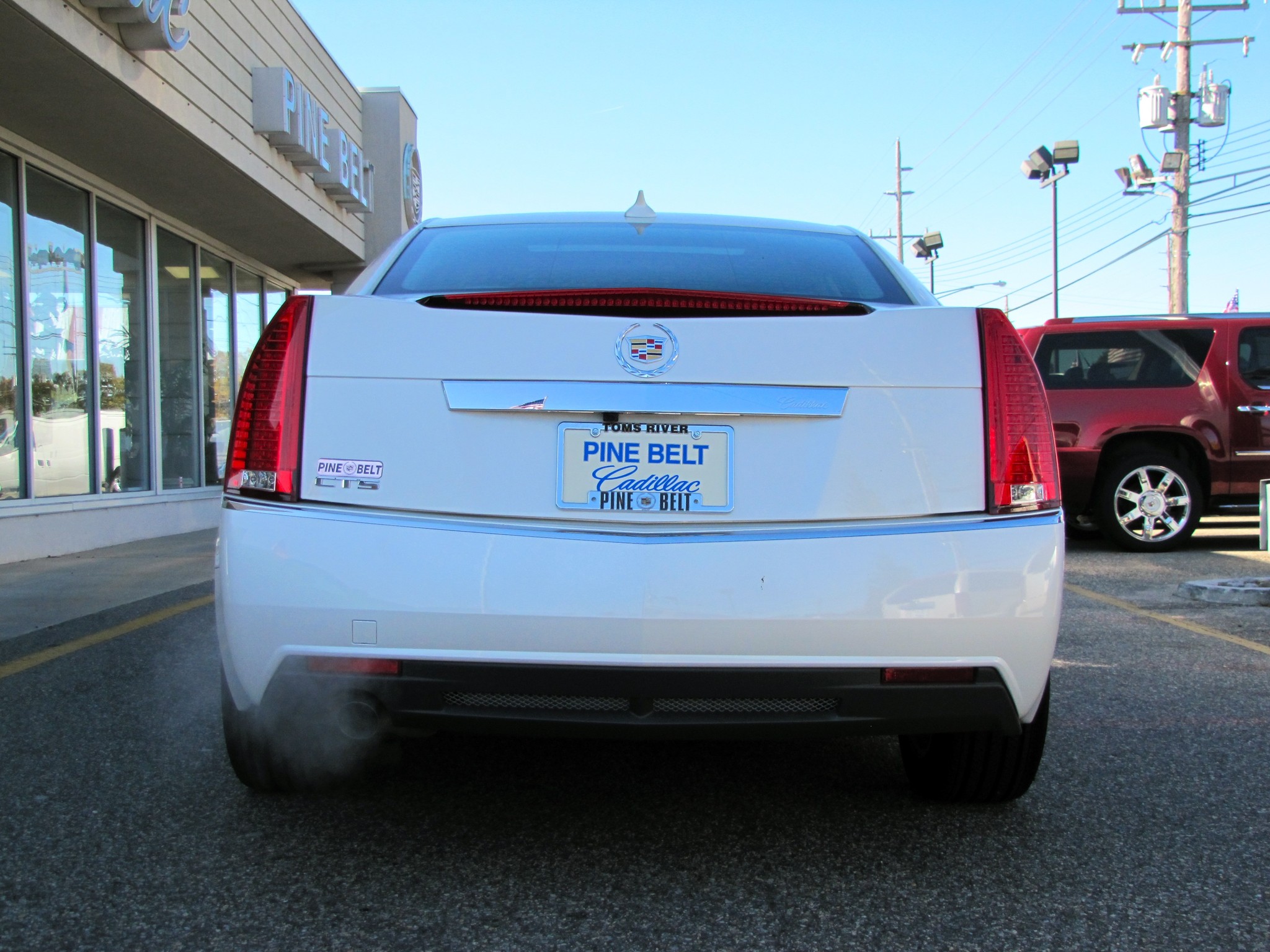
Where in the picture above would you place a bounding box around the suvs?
[1013,313,1270,551]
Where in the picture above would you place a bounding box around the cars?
[215,187,1068,806]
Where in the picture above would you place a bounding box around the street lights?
[1021,140,1082,317]
[1116,149,1192,319]
[911,230,942,295]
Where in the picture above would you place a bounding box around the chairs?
[1063,366,1085,388]
[1086,361,1112,388]
